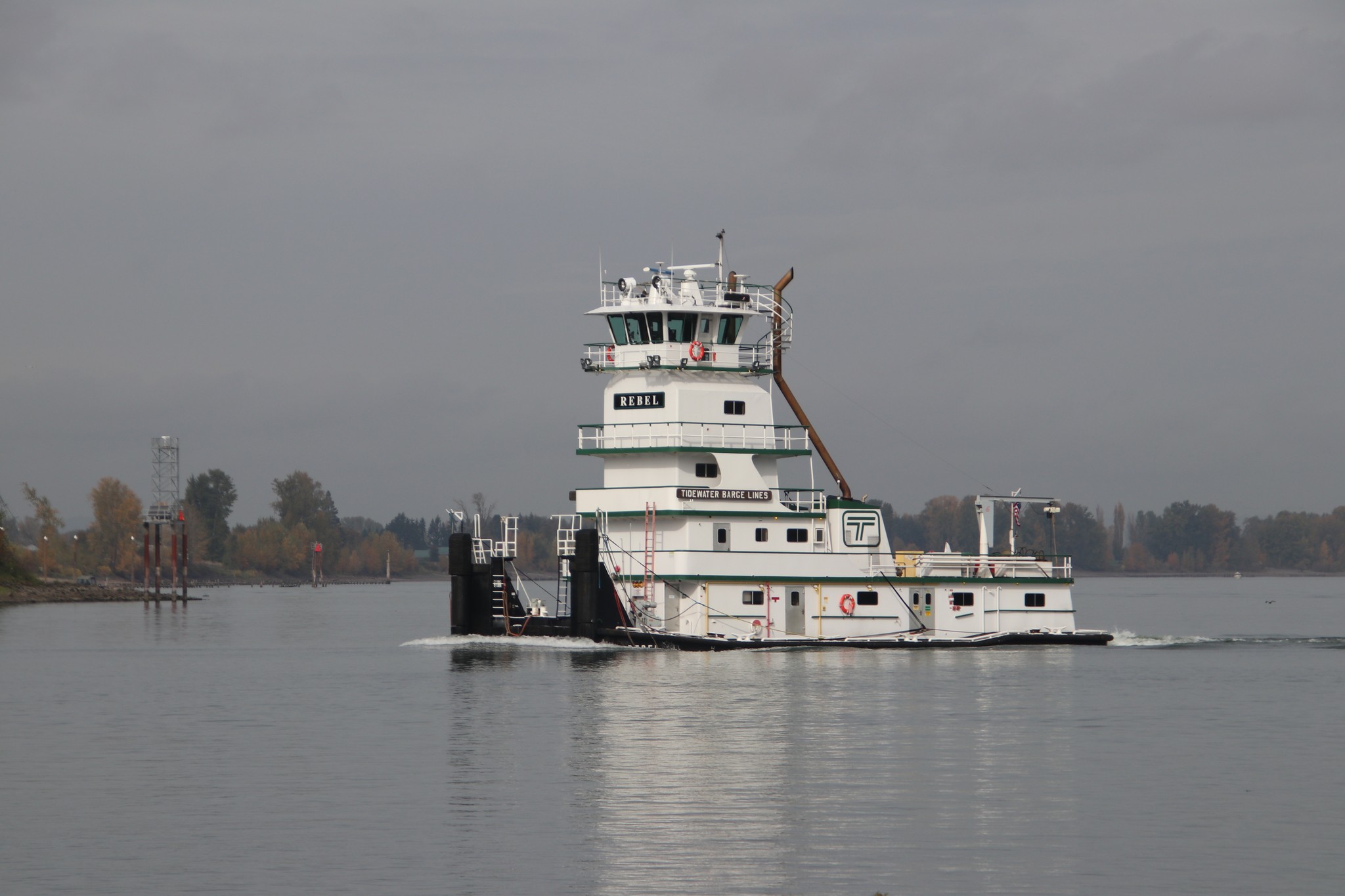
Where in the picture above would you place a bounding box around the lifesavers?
[689,341,704,360]
[607,345,615,361]
[840,594,854,613]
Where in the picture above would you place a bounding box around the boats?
[447,224,1118,649]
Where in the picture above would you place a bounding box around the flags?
[1013,500,1021,526]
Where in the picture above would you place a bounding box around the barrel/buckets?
[526,605,547,616]
